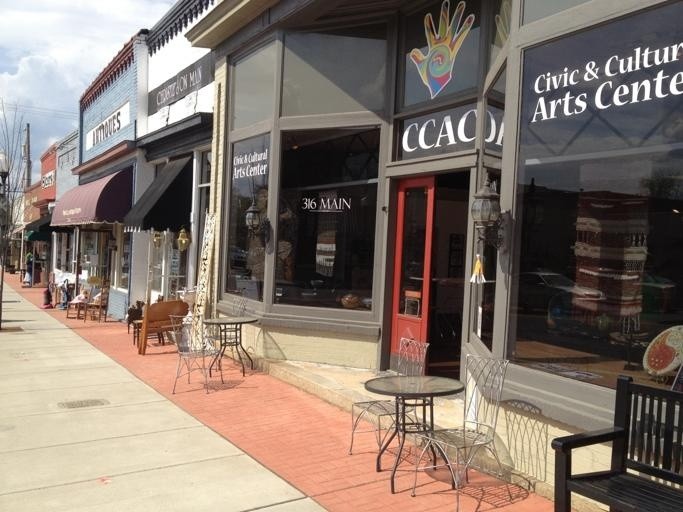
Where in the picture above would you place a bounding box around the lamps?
[468,171,505,252]
[174,226,190,253]
[107,233,117,252]
[244,195,270,243]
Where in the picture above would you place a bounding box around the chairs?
[65,280,110,324]
[551,375,681,511]
[348,337,514,512]
[167,313,223,396]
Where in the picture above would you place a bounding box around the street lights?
[1,148,12,329]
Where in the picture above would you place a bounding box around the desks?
[202,317,258,379]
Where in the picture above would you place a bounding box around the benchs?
[135,299,188,354]
